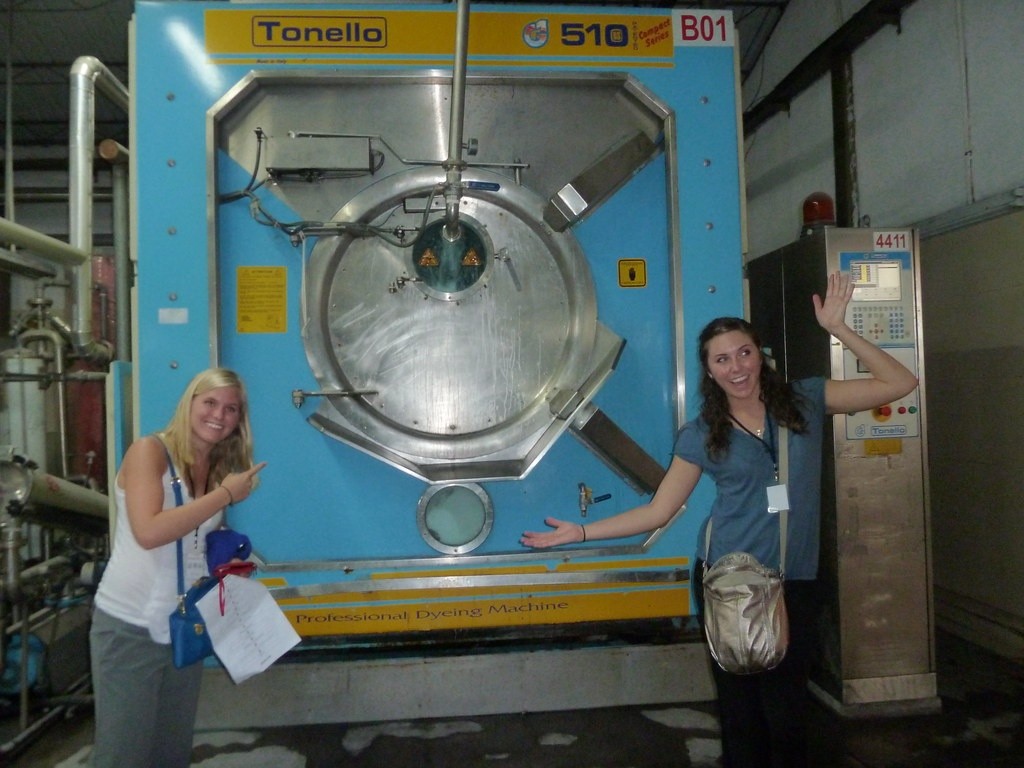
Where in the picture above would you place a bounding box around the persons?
[89,369,265,768]
[519,271,918,768]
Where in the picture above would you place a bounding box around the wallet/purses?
[214,561,258,616]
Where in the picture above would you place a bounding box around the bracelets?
[221,486,234,506]
[581,525,586,542]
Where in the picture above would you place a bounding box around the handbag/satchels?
[701,551,790,674]
[169,576,215,668]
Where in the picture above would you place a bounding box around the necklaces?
[734,413,765,437]
